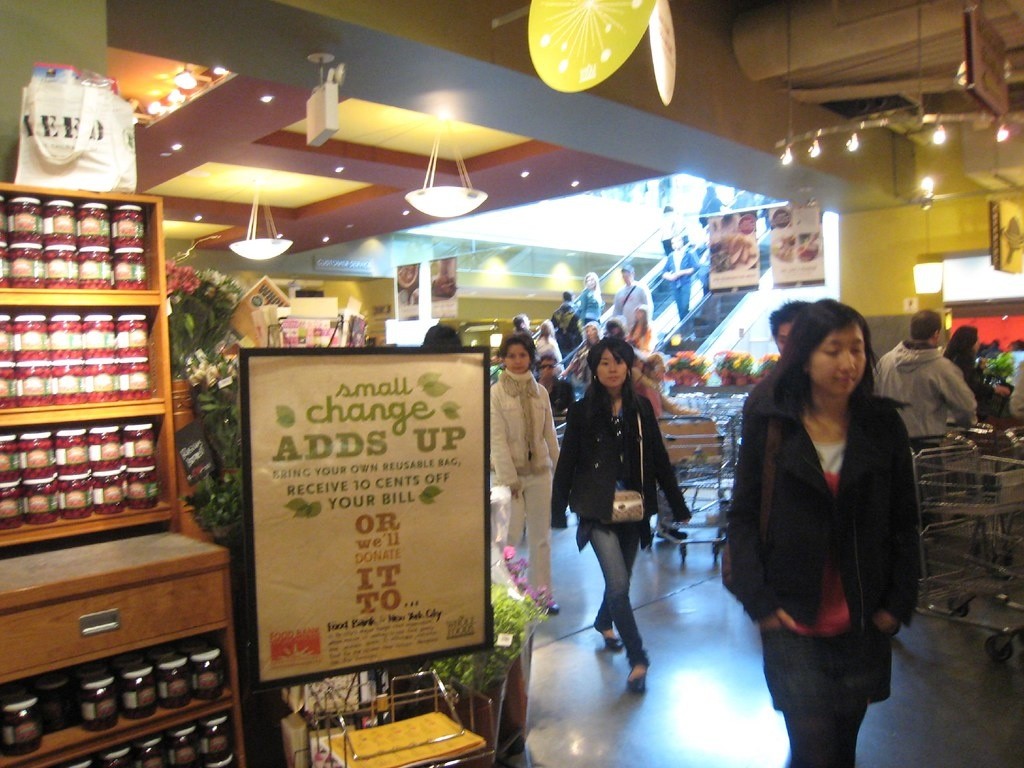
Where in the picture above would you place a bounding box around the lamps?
[228,182,294,261]
[405,119,488,217]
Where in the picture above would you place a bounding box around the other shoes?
[545,600,562,614]
[626,664,645,694]
[594,623,625,650]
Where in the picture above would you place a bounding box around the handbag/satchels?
[721,539,741,595]
[600,488,644,524]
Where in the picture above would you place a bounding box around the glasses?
[542,363,557,369]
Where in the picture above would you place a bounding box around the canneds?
[0,638,236,768]
[0,195,161,530]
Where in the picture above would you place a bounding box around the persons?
[515,264,698,542]
[943,325,1024,421]
[727,298,921,768]
[551,337,692,694]
[490,336,561,615]
[659,185,780,322]
[422,324,461,347]
[872,309,978,590]
[768,301,813,356]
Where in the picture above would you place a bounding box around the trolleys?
[644,389,755,566]
[903,418,1024,661]
